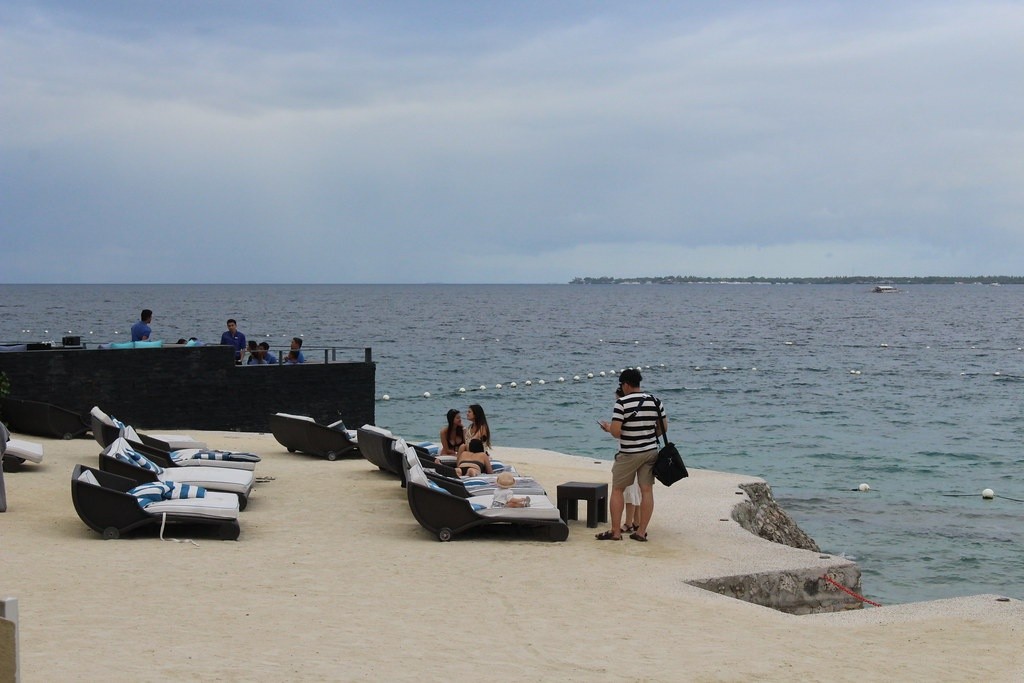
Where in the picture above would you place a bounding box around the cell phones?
[597,421,605,429]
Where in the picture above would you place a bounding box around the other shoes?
[526,496,531,507]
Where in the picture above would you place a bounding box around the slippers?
[630,532,648,541]
[595,531,623,540]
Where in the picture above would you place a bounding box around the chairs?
[269,412,570,541]
[71,407,262,540]
[2,438,44,471]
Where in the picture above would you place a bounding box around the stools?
[556,481,608,528]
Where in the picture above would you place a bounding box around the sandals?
[632,522,639,531]
[620,524,633,533]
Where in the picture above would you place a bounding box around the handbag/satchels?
[652,442,688,487]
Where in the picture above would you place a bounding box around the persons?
[465,404,492,455]
[440,408,466,456]
[594,368,668,541]
[247,337,304,365]
[176,337,198,344]
[220,318,246,365]
[131,309,152,341]
[434,438,493,477]
[490,471,531,507]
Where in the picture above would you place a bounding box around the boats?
[874,285,899,293]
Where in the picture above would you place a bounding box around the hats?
[496,472,516,488]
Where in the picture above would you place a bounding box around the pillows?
[97,338,206,349]
